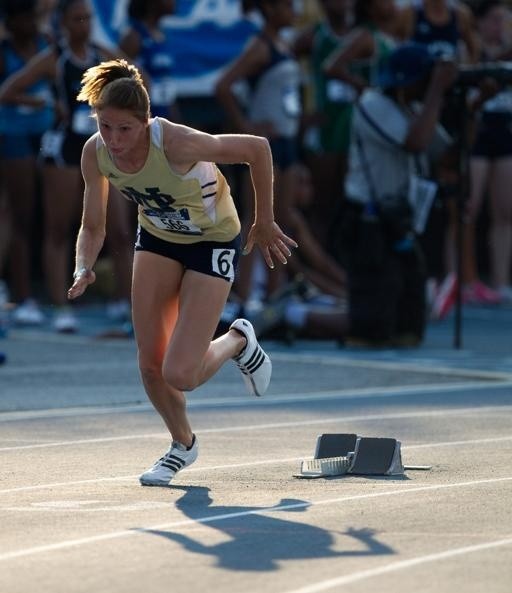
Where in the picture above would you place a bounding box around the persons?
[69,59,299,485]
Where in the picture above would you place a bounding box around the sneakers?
[0,299,137,366]
[424,272,511,322]
[229,317,273,398]
[138,433,200,488]
[223,284,344,319]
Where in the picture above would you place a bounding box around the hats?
[377,42,434,90]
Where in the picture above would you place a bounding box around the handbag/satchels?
[377,185,449,275]
